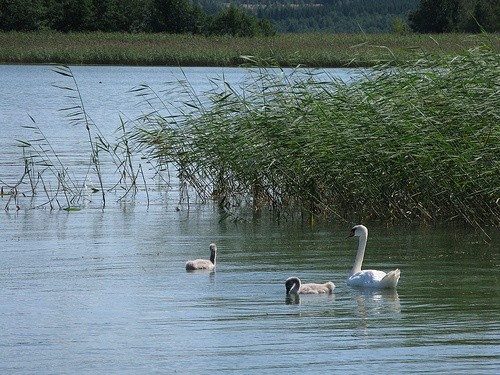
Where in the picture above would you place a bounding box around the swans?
[345,224,401,288]
[286,277,335,295]
[185,243,217,272]
